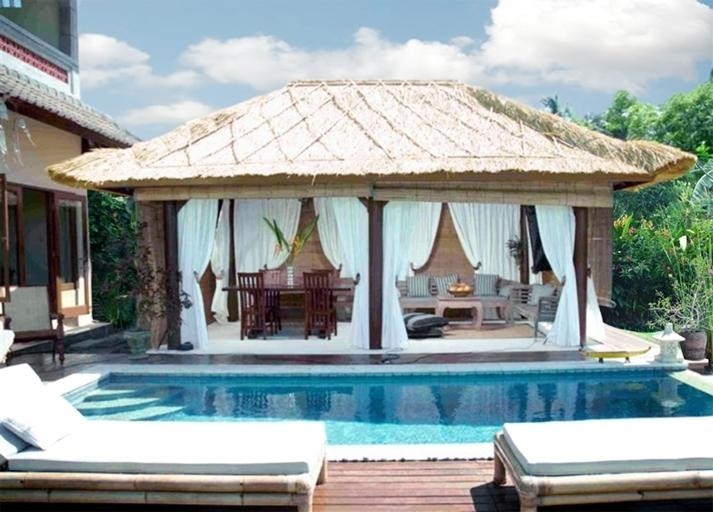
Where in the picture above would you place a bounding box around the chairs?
[1,282,66,365]
[237,264,344,340]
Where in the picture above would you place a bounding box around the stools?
[579,344,651,363]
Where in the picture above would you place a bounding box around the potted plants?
[505,232,528,266]
[645,279,709,360]
[262,213,321,286]
[97,220,192,359]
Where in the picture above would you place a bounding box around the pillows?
[0,361,84,450]
[403,272,501,299]
[530,281,557,306]
[402,312,449,339]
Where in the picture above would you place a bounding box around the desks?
[435,295,484,331]
[221,277,354,339]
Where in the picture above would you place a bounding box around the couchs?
[396,278,516,322]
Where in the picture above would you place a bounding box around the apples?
[449,283,470,291]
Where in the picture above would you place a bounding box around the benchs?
[493,413,711,511]
[507,281,563,338]
[0,364,328,512]
